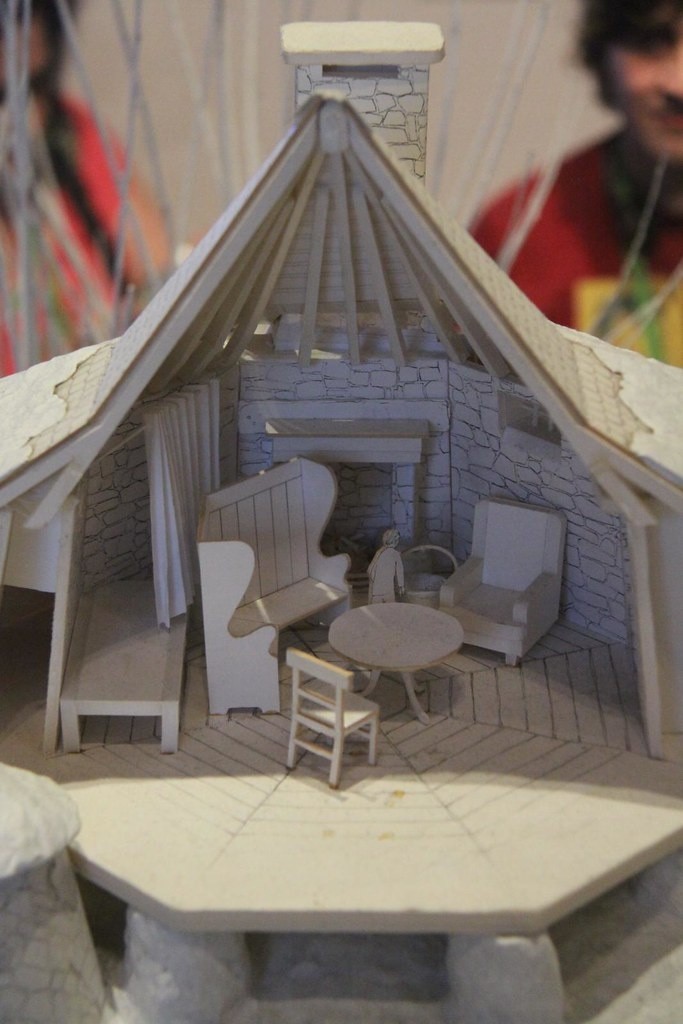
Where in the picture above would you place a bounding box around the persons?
[467,0,683,370]
[0,0,175,378]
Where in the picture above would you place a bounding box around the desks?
[326,602,464,726]
[205,576,355,715]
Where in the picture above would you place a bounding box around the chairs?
[283,651,382,790]
[438,496,568,665]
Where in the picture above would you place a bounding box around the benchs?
[60,577,188,754]
